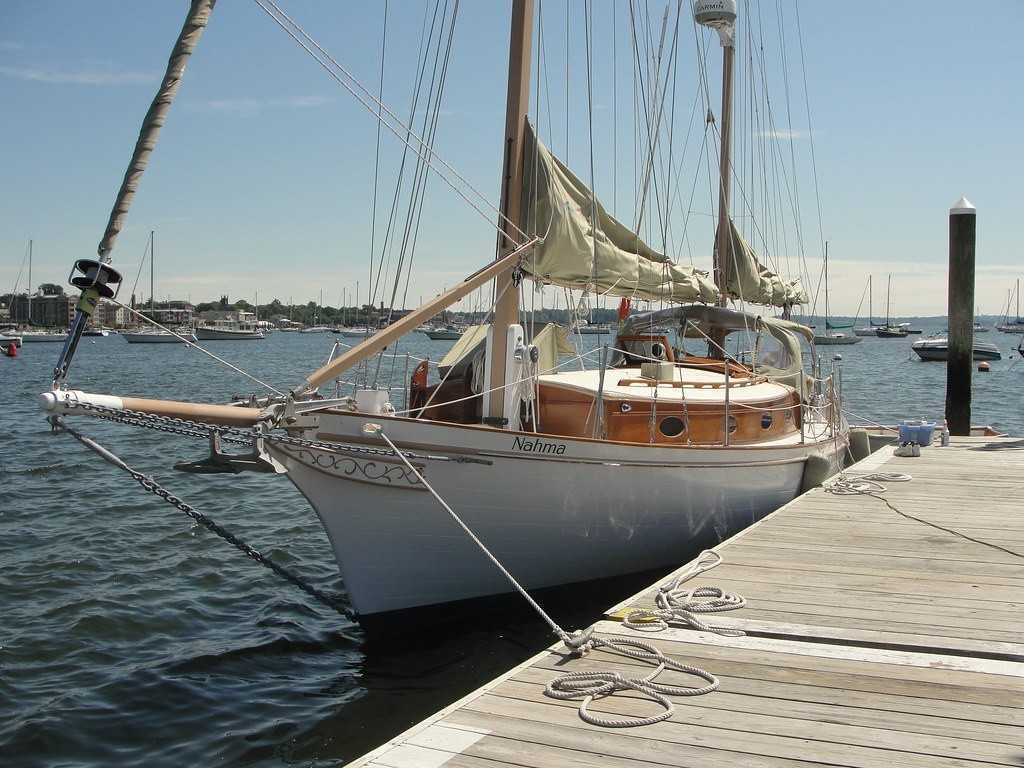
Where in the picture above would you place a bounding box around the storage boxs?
[642,362,675,381]
[897,422,936,445]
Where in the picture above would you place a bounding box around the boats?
[910,326,1003,361]
[411,281,492,340]
[643,325,670,334]
[800,322,817,329]
[572,321,611,335]
[0,333,24,348]
[193,323,265,339]
[1011,335,1024,359]
[80,324,110,337]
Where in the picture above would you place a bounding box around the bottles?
[941,420,950,446]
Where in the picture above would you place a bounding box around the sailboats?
[38,0,856,666]
[252,278,386,337]
[993,276,1024,333]
[973,306,990,333]
[810,241,861,345]
[850,272,924,338]
[118,230,199,342]
[0,239,70,342]
